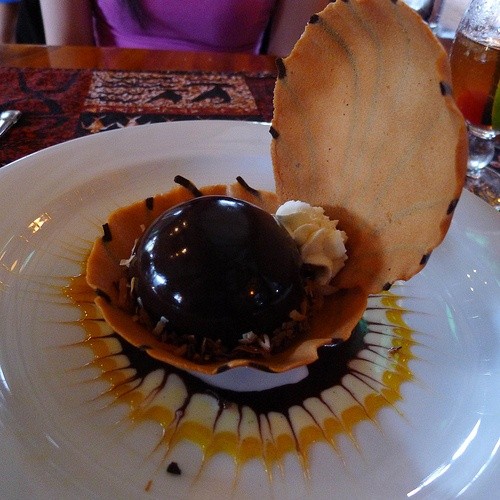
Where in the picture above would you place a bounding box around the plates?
[2,120,500,500]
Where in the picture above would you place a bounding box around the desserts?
[121,193,350,358]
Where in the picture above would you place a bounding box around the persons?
[39,0,333,58]
[0,0,44,44]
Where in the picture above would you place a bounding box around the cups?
[449,0,500,140]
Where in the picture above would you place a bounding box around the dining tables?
[2,45,500,211]
